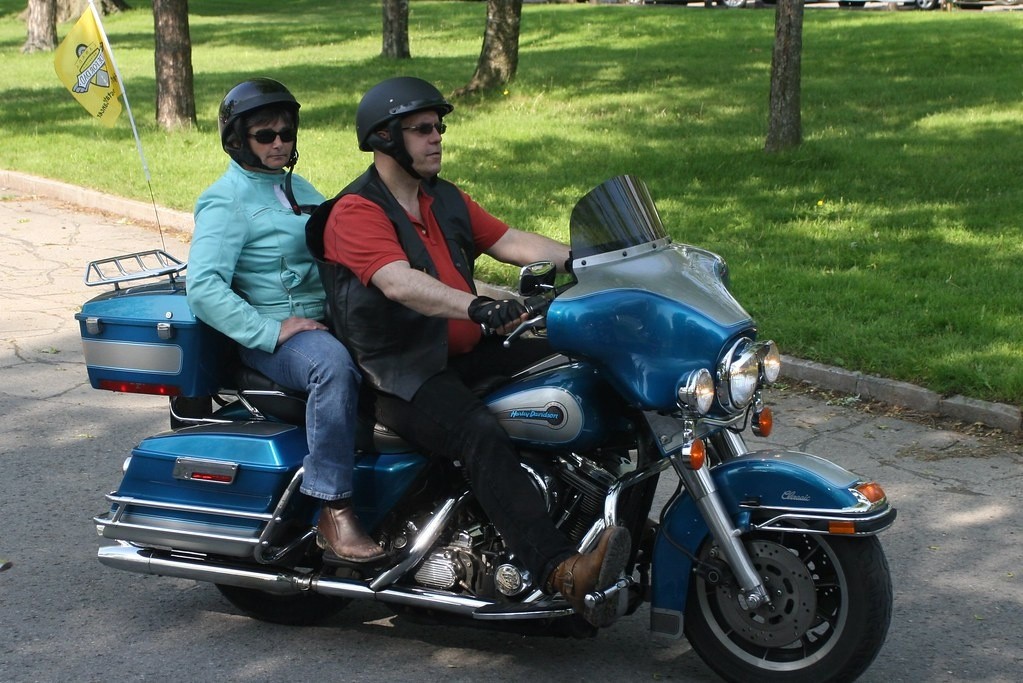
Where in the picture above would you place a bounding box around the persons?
[183,77,387,564]
[308,75,631,631]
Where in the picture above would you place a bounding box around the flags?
[52,4,123,129]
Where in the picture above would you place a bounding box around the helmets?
[356,77,455,151]
[218,78,301,153]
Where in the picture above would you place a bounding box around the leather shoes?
[316,505,385,563]
[553,526,631,628]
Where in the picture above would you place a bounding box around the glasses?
[384,122,448,135]
[247,126,296,144]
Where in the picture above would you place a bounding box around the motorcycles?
[74,171,898,682]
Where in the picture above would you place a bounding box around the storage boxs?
[106,419,311,559]
[73,275,229,399]
